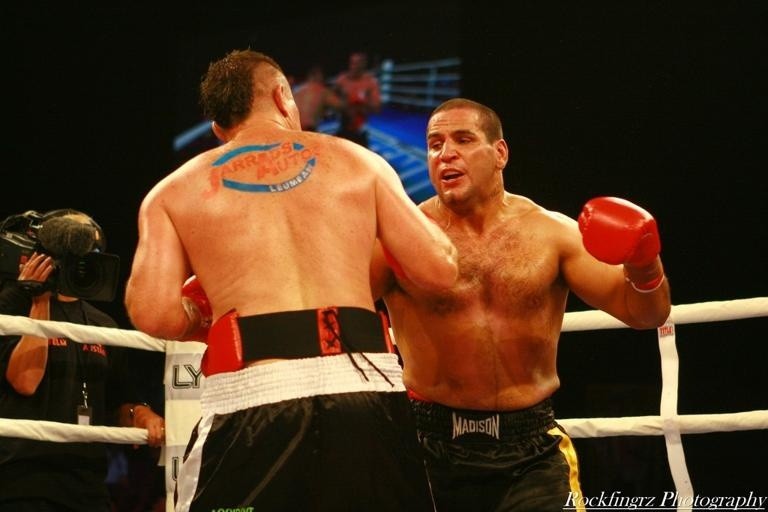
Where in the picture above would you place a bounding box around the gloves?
[347,89,369,106]
[578,197,666,294]
[178,273,213,346]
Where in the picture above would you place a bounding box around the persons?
[0,207,167,511]
[123,50,461,510]
[331,53,383,147]
[290,66,356,133]
[369,99,674,512]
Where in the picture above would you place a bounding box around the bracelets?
[126,403,151,418]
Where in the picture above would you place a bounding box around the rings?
[162,427,166,430]
